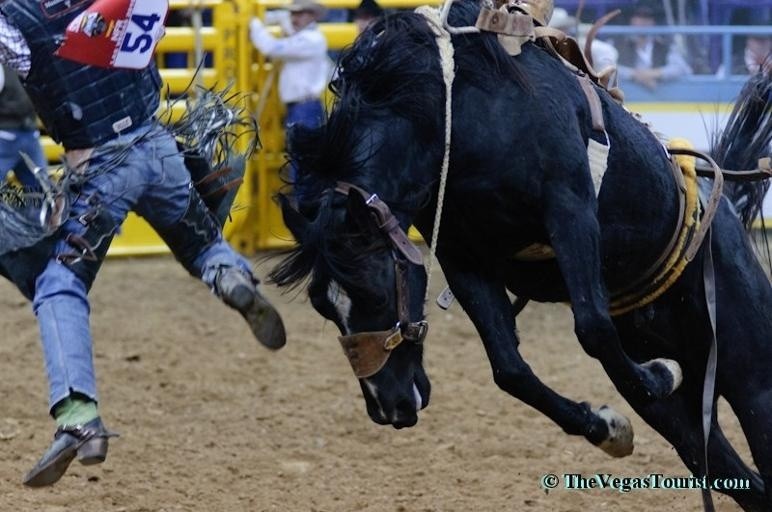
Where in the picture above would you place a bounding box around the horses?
[252,0,772,512]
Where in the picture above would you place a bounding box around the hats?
[289,0,326,20]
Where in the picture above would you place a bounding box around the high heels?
[23,417,120,487]
[216,268,286,349]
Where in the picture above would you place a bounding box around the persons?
[347,1,384,38]
[0,62,60,307]
[0,0,286,488]
[604,7,693,92]
[744,35,772,74]
[248,0,333,202]
[546,7,619,76]
